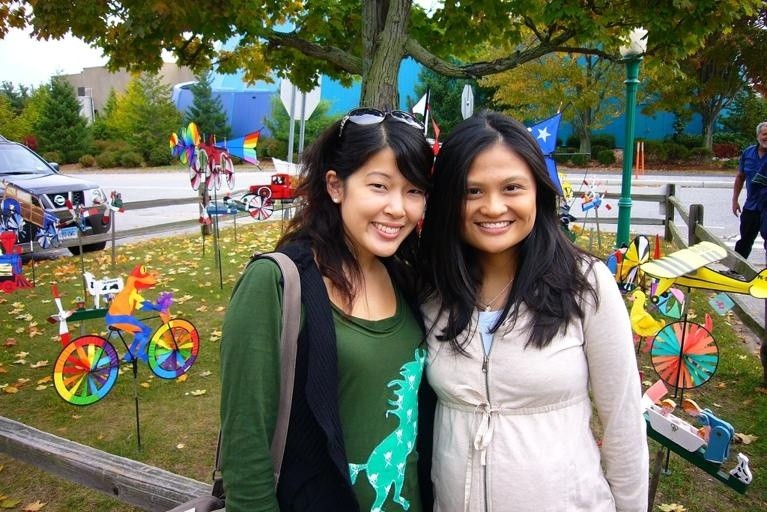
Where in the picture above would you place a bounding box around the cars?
[0,135,111,255]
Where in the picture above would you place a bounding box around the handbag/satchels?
[163,494,226,512]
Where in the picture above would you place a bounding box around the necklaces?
[482,278,513,313]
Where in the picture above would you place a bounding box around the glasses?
[338,106,425,138]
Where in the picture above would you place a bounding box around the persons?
[216,108,426,511]
[414,112,649,512]
[718,122,766,280]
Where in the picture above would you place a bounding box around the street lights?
[614,26,648,249]
